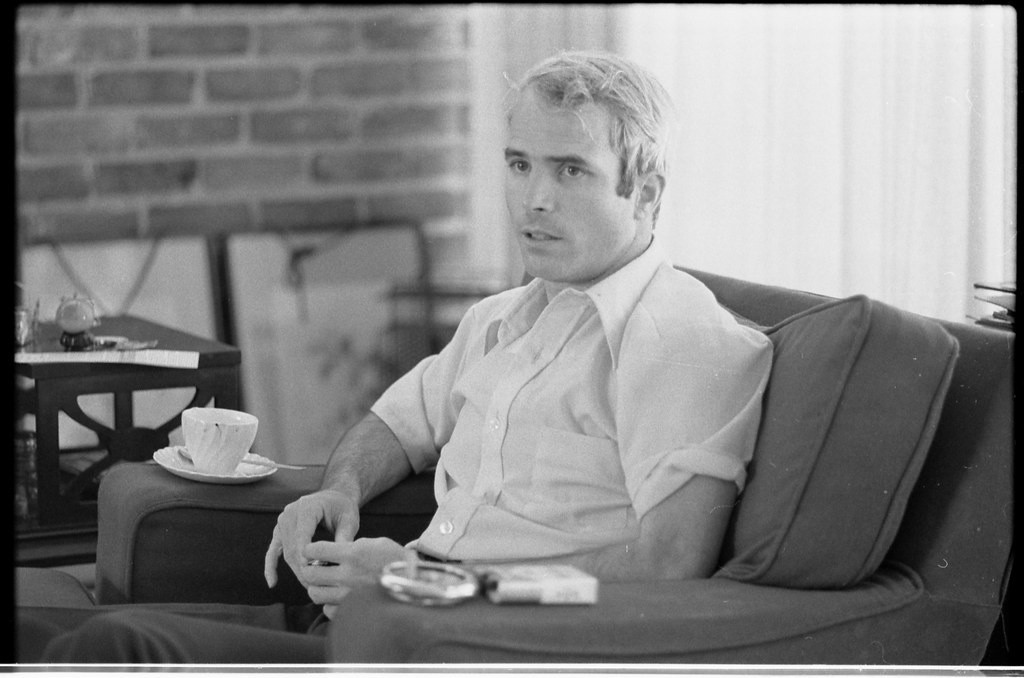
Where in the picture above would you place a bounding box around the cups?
[181,407,259,477]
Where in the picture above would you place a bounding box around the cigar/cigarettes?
[406,550,418,581]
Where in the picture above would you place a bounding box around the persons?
[40,50,776,662]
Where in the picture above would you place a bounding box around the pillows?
[716,294,963,588]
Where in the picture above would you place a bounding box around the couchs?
[95,265,1024,668]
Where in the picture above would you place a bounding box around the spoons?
[179,447,306,470]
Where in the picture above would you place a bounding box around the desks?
[12,314,244,566]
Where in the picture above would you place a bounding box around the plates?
[153,446,278,484]
